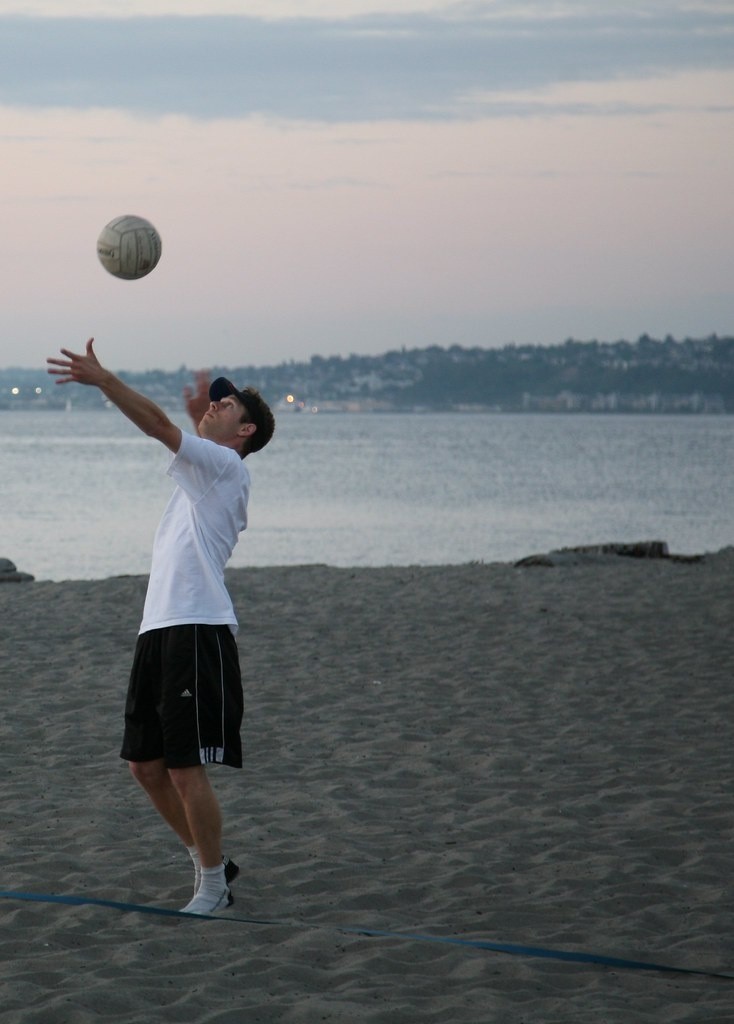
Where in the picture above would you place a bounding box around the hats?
[209,374,265,454]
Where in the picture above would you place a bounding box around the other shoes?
[179,855,239,915]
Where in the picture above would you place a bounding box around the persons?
[45,337,276,914]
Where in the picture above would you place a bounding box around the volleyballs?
[95,213,162,281]
